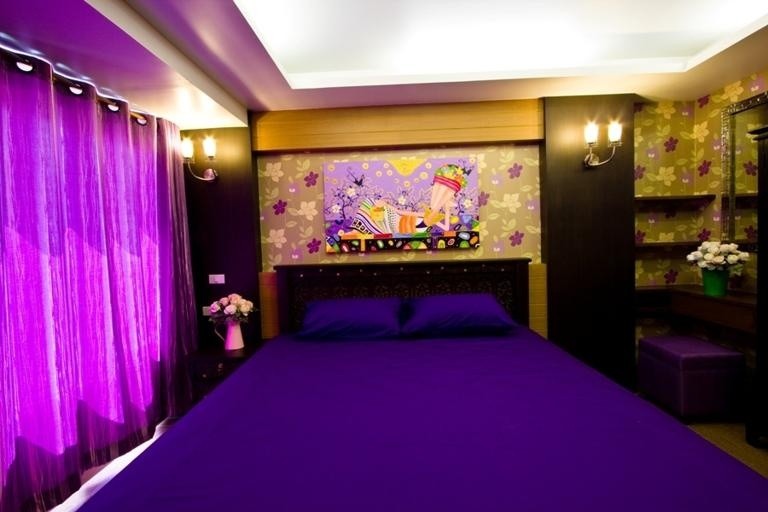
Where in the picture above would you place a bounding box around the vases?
[213,319,245,351]
[700,265,731,297]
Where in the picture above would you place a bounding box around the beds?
[49,256,768,511]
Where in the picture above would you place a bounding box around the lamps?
[175,133,221,183]
[583,122,624,168]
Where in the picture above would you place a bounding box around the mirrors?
[719,91,767,295]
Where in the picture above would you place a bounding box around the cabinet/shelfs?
[634,192,716,257]
[734,190,764,255]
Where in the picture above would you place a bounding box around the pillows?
[292,291,520,344]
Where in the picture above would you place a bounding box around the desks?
[636,282,757,334]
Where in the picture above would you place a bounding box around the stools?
[639,334,747,423]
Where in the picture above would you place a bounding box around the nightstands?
[189,337,271,405]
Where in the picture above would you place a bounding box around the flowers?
[686,240,739,280]
[204,293,255,325]
[728,243,752,278]
